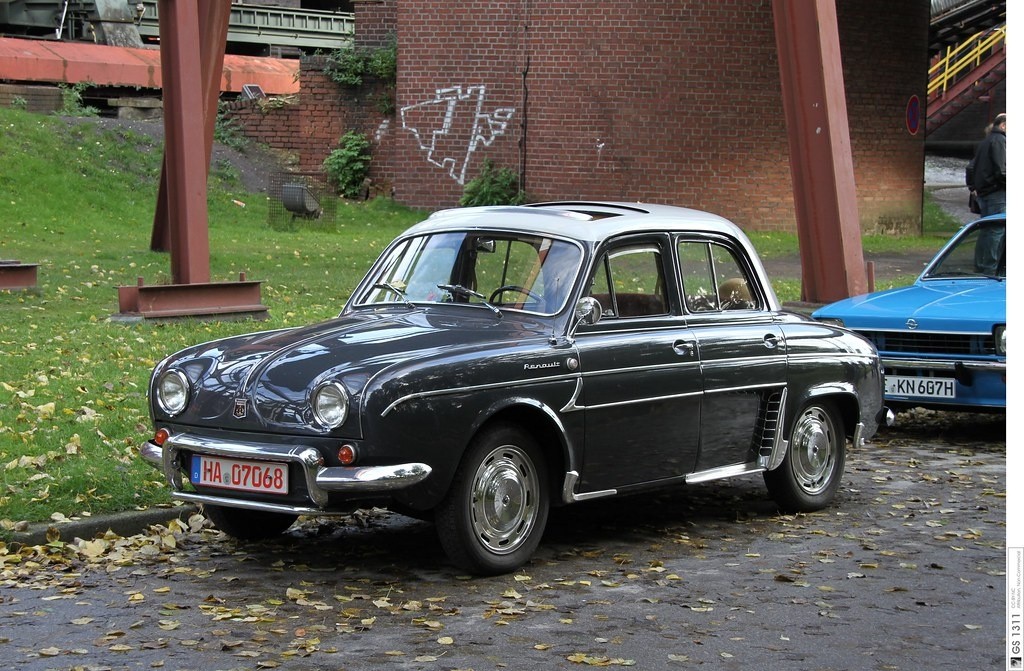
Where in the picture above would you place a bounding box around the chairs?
[591,292,750,316]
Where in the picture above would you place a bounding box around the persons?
[965,111,1006,275]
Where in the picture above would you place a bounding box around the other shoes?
[973,268,984,273]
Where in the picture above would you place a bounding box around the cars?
[812,210,1005,416]
[148,198,886,577]
